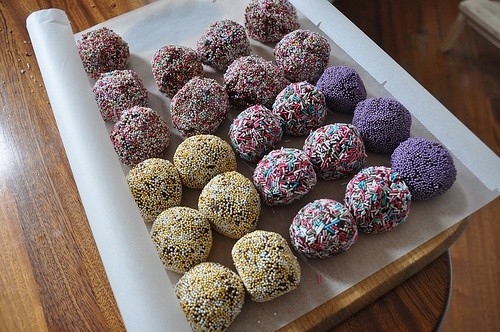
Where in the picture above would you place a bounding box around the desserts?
[76,1,457,331]
